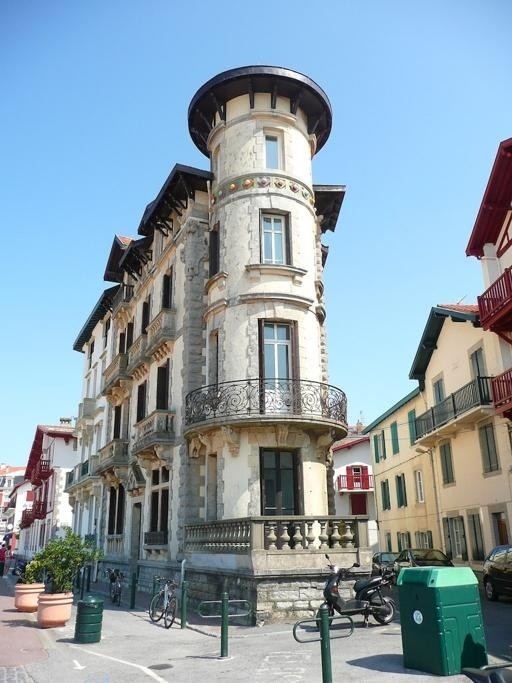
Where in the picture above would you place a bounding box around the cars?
[483,545,512,600]
[371,549,454,585]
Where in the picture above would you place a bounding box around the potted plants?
[13,525,84,611]
[36,534,103,626]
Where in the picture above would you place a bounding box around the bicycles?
[106,568,123,606]
[149,576,179,628]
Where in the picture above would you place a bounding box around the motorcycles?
[316,554,396,627]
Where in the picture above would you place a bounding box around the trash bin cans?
[396,566,488,675]
[75,596,104,643]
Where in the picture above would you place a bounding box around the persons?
[0,542,13,579]
